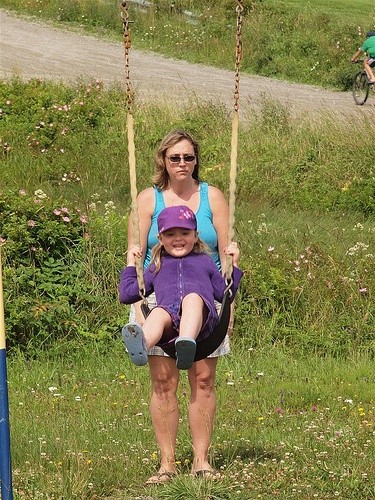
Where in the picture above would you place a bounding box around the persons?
[119,206,244,369]
[127,132,234,483]
[351,32,375,84]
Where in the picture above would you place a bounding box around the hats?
[157,205,197,233]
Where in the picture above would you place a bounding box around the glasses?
[164,154,195,162]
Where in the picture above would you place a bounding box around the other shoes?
[175,337,196,369]
[122,323,148,366]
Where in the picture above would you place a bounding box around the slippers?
[196,470,222,479]
[145,472,174,484]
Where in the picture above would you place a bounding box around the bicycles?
[351,59,375,106]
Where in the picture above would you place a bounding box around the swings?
[120,0,244,362]
